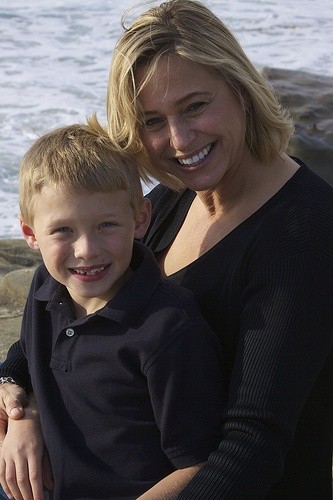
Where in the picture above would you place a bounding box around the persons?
[1,121,232,500]
[1,0,331,500]
